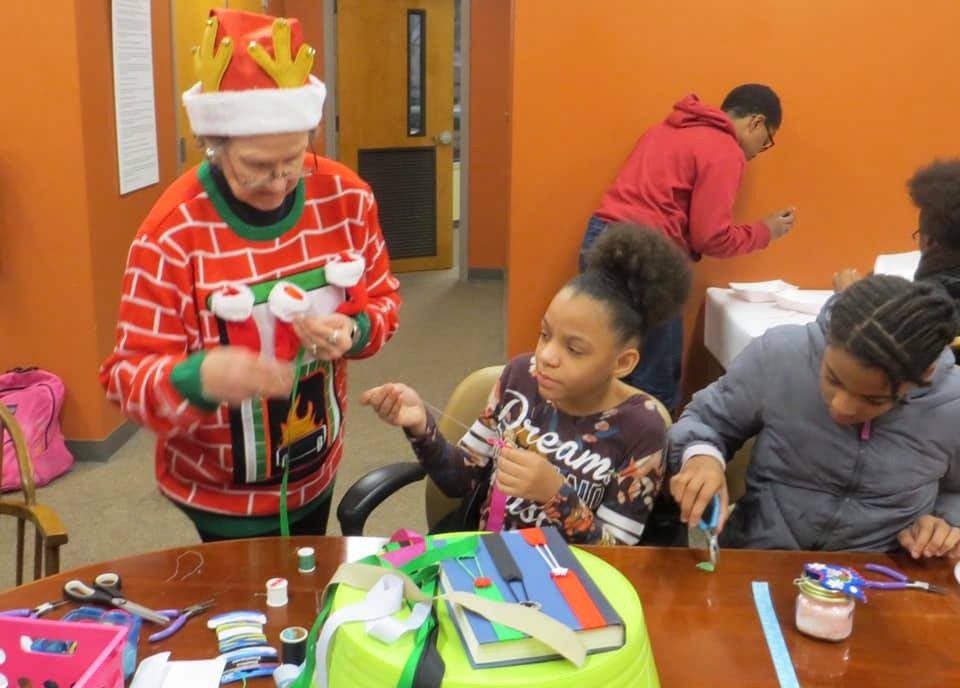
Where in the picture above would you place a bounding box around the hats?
[182,7,327,138]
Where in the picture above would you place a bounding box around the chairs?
[0,403,67,594]
[339,359,676,540]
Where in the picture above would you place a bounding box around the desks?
[0,532,960,688]
[706,287,834,369]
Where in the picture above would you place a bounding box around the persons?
[99,5,404,546]
[576,81,796,410]
[664,273,960,559]
[833,161,960,364]
[362,222,695,546]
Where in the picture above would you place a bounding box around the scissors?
[63,573,171,627]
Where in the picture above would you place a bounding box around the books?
[430,525,627,671]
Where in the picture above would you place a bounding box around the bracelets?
[354,323,361,343]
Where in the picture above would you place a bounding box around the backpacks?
[0,365,74,494]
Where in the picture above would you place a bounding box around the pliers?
[147,600,217,643]
[865,563,948,595]
[699,493,721,563]
[0,598,68,619]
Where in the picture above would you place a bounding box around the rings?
[310,344,317,355]
[328,331,336,344]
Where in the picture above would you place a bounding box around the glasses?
[220,137,319,190]
[912,227,922,244]
[751,113,776,153]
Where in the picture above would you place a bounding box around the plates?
[775,289,834,316]
[728,279,798,302]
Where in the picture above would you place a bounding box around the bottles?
[796,572,853,640]
